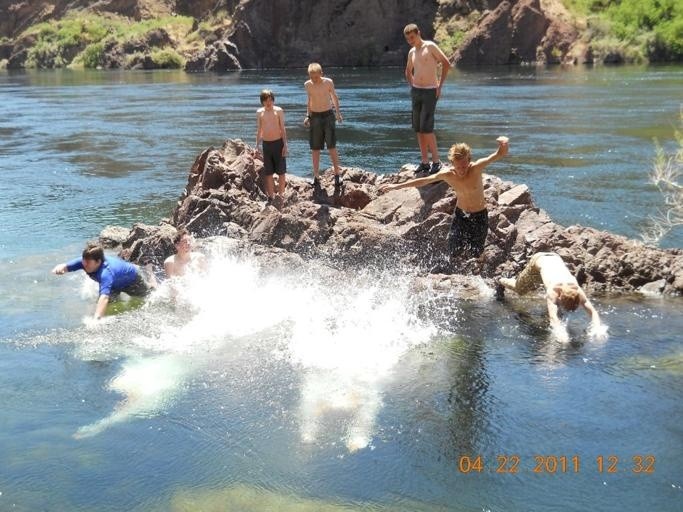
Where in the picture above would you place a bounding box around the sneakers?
[335,175,344,185]
[430,161,442,174]
[414,162,431,172]
[314,177,321,186]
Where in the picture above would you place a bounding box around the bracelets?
[306,114,311,119]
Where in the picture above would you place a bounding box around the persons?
[164,230,202,282]
[254,89,290,199]
[302,62,345,187]
[402,22,452,176]
[377,135,512,263]
[494,251,606,346]
[52,244,156,319]
